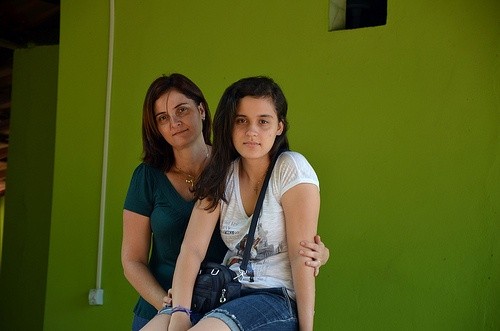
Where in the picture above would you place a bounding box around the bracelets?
[171,305,192,316]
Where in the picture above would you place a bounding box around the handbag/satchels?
[189,261,241,311]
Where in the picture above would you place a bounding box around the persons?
[122,73,329,331]
[136,76,320,331]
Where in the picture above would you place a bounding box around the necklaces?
[175,145,210,185]
[253,168,267,195]
